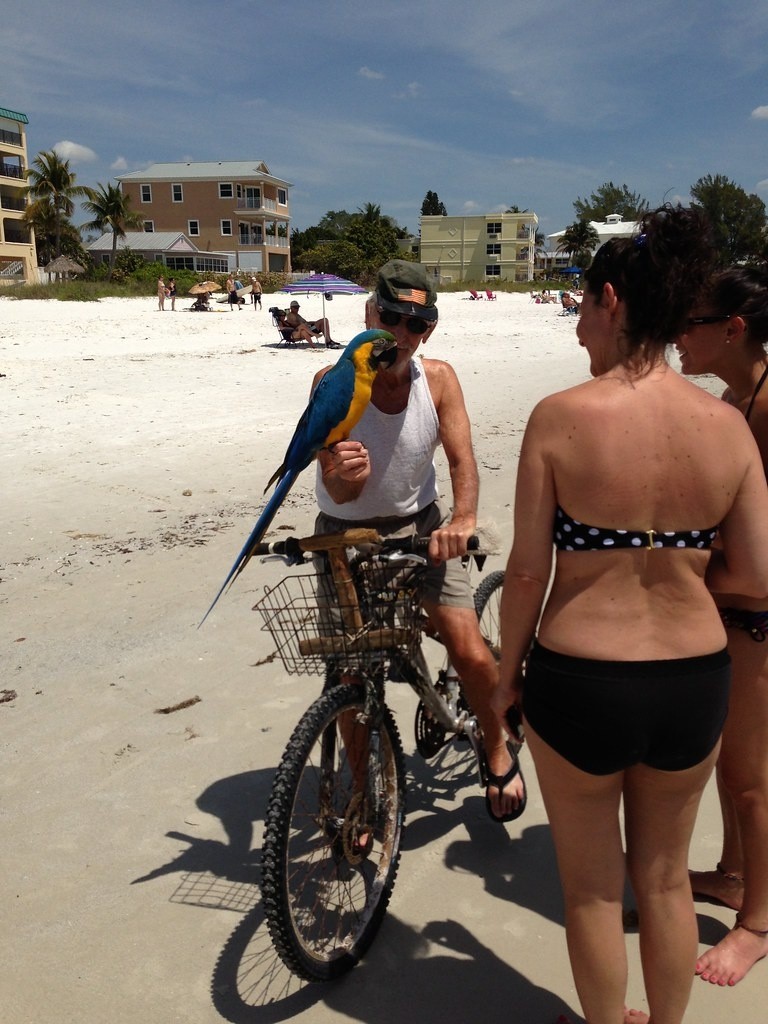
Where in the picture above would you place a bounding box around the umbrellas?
[559,267,583,279]
[188,280,222,295]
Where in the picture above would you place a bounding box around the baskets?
[251,559,427,676]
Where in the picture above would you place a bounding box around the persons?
[276,272,369,347]
[561,292,582,315]
[530,288,556,304]
[226,274,243,310]
[191,293,211,310]
[496,187,768,1024]
[676,261,766,987]
[248,275,263,311]
[570,277,583,296]
[158,274,177,311]
[311,260,527,858]
[278,301,340,349]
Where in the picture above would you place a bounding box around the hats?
[291,301,300,307]
[252,277,257,281]
[374,259,438,322]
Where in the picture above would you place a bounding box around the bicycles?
[245,526,535,984]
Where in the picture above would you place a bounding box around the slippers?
[480,738,527,821]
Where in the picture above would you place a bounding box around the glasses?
[373,299,432,334]
[280,315,286,317]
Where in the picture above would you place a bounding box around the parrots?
[196,328,398,629]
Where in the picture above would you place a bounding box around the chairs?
[269,307,306,348]
[470,286,577,316]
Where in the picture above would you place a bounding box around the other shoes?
[327,340,340,349]
